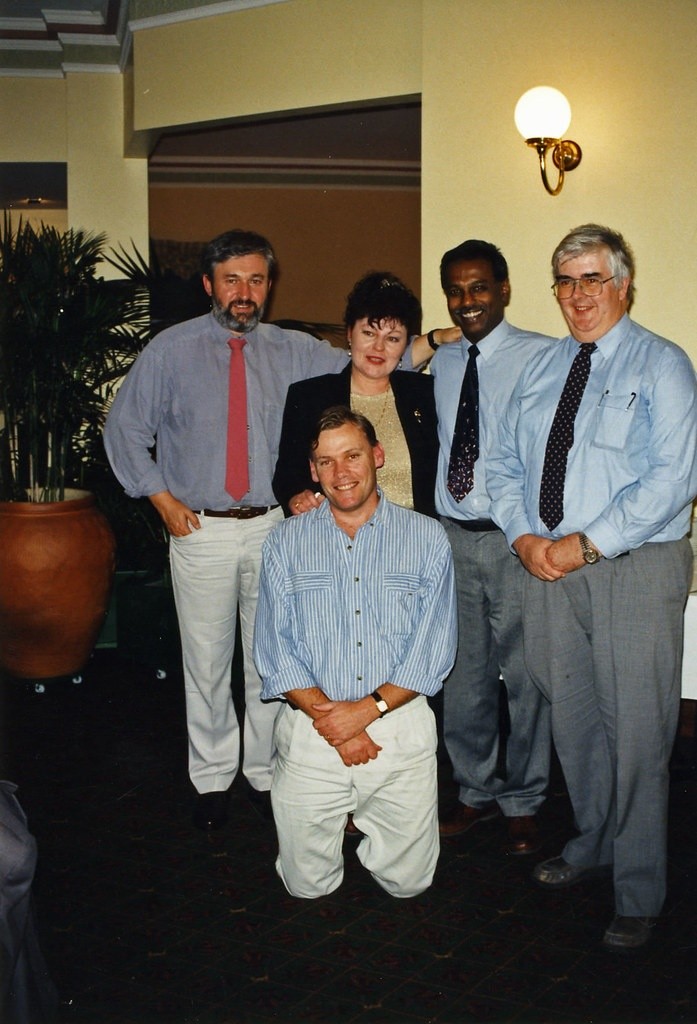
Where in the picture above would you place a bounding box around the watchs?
[579,534,600,564]
[371,692,389,718]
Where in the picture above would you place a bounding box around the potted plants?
[0,207,214,680]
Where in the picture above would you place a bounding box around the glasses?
[551,273,619,300]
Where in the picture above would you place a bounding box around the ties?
[539,341,598,532]
[447,342,480,504]
[224,337,250,503]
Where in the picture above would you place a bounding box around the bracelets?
[428,329,439,350]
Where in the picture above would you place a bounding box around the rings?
[327,735,330,739]
[295,503,299,507]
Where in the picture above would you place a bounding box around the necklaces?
[351,384,390,427]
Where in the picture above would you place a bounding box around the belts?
[447,516,501,532]
[192,503,280,519]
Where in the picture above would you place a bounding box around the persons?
[481,222,697,949]
[428,239,561,859]
[270,272,443,525]
[103,228,466,846]
[253,407,458,898]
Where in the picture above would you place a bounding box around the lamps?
[515,86,583,199]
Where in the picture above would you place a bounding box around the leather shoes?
[343,813,362,836]
[439,801,499,838]
[504,814,544,856]
[603,912,660,952]
[198,785,232,832]
[530,855,614,890]
[240,773,274,818]
